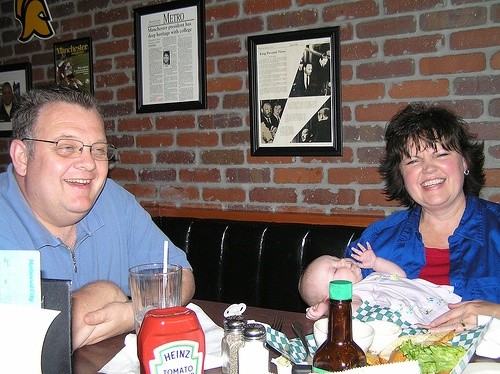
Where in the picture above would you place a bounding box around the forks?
[270,316,286,332]
[292,321,313,364]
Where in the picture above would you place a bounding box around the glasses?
[21,138,118,161]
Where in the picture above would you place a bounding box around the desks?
[71,305,315,374]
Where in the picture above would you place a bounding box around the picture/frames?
[133,0,209,115]
[246,24,345,157]
[0,61,33,138]
[54,36,94,94]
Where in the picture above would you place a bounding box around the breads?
[386,346,451,374]
[366,350,381,365]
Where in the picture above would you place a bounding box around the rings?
[459,318,463,324]
[461,323,466,331]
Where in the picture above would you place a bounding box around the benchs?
[149,202,383,314]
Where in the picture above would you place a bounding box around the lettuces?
[395,339,466,374]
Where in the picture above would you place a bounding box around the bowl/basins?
[365,319,402,354]
[312,317,376,353]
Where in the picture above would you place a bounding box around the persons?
[300,107,331,143]
[0,82,196,355]
[290,62,317,96]
[59,61,84,89]
[0,82,21,122]
[163,51,170,65]
[261,102,282,143]
[341,102,500,334]
[298,241,500,360]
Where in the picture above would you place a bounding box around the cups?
[128,263,183,336]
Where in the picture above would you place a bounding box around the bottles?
[136,306,206,374]
[238,322,270,374]
[311,281,367,374]
[221,315,247,374]
